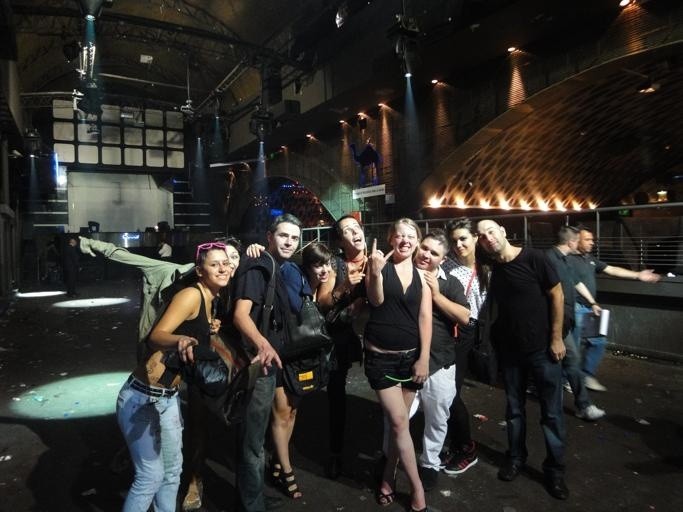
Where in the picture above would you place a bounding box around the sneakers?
[418,440,479,492]
[581,370,607,392]
[574,404,607,421]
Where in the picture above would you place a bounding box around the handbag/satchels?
[264,262,335,365]
[281,346,336,397]
[182,325,258,443]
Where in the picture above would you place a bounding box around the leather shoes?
[497,448,528,481]
[541,457,570,500]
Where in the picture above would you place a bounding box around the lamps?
[401,47,412,77]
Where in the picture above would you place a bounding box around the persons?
[116,214,662,512]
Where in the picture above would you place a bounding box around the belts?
[363,345,418,361]
[127,372,180,397]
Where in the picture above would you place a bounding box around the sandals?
[271,460,303,499]
[378,489,396,507]
[180,474,207,511]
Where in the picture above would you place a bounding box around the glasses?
[194,241,227,263]
